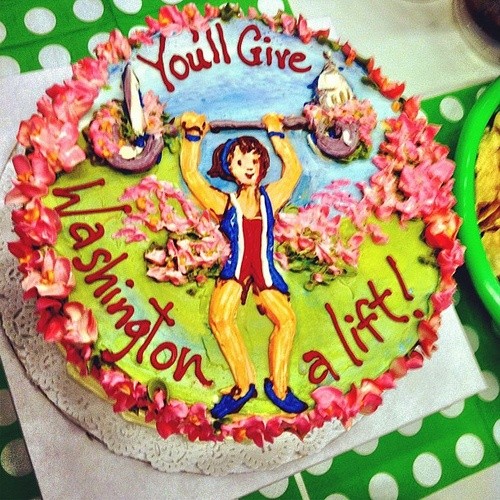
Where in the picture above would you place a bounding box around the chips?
[473,111,500,276]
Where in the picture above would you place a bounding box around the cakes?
[3,4,460,448]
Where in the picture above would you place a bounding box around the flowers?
[5,1,468,446]
